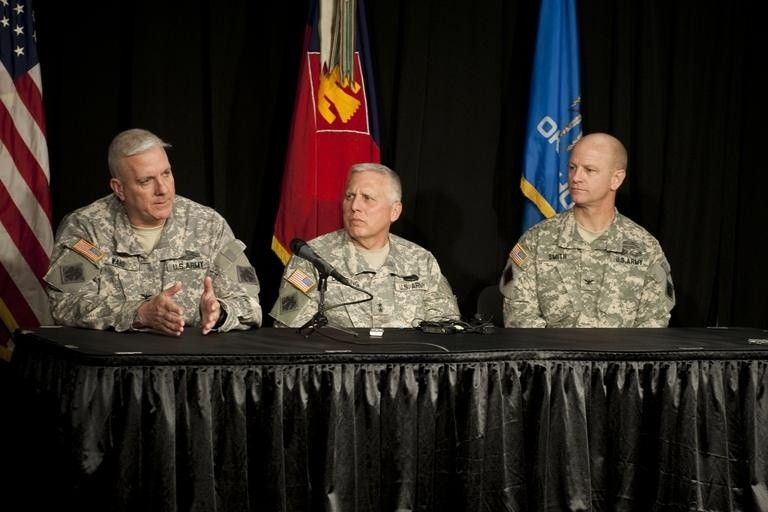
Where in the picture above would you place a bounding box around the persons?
[268,163,462,328]
[42,128,262,339]
[497,132,676,330]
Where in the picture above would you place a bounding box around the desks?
[11,321,768,507]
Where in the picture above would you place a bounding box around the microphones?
[288,238,350,286]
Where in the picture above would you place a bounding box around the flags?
[269,0,380,267]
[0,0,56,363]
[519,0,583,236]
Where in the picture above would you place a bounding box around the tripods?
[296,277,359,340]
